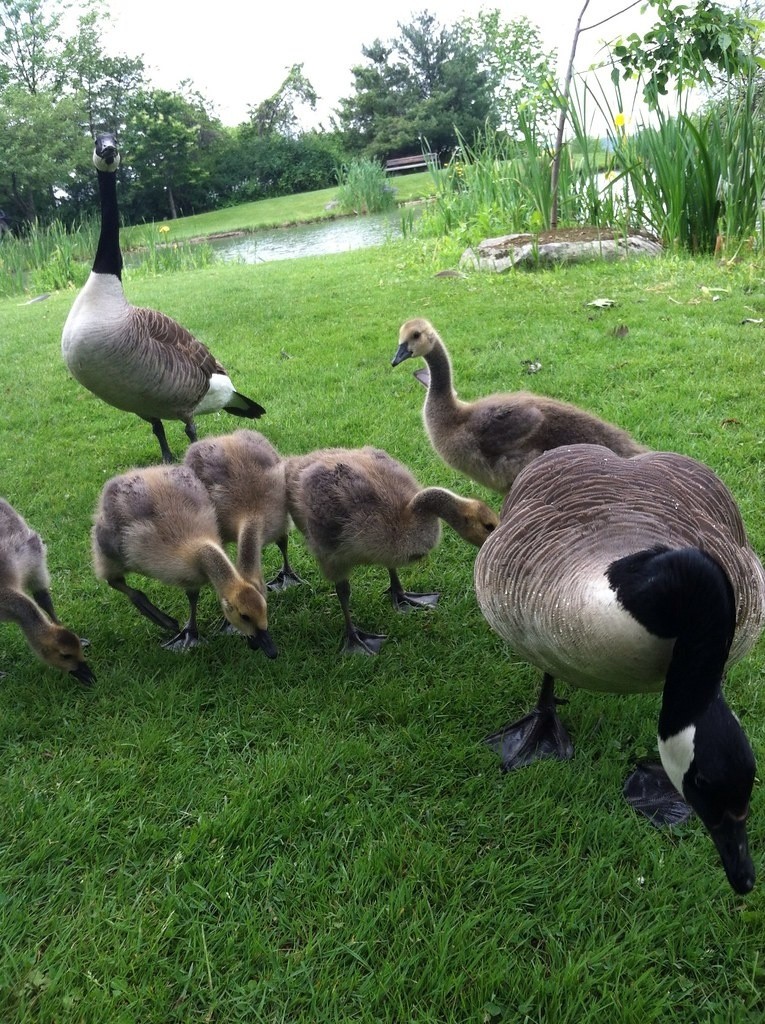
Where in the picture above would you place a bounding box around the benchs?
[386,153,442,164]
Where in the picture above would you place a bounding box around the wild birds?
[473,442,765,897]
[391,319,650,499]
[89,462,277,659]
[0,495,98,688]
[61,133,267,466]
[283,444,499,657]
[182,428,305,651]
